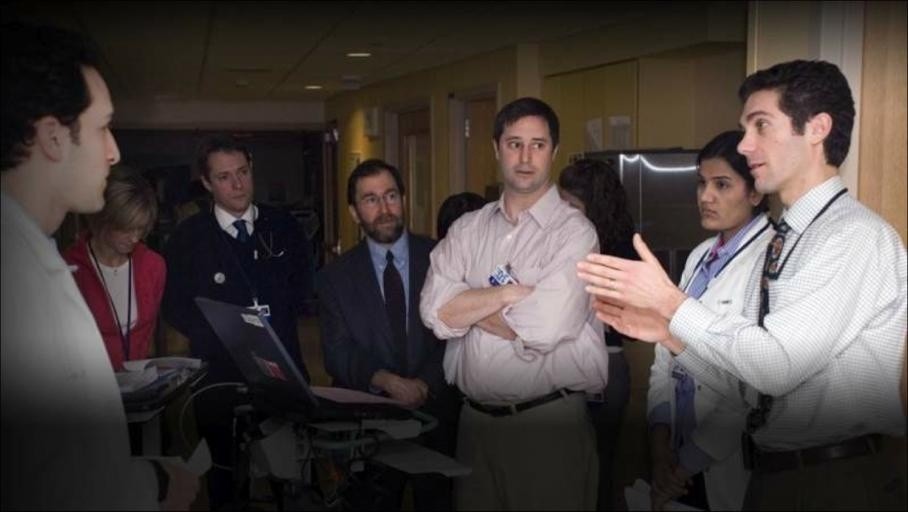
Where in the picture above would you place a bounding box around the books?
[115,356,210,412]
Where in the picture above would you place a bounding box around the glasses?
[354,192,403,203]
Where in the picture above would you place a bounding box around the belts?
[462,386,578,416]
[748,433,896,467]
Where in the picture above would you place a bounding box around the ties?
[382,250,406,350]
[746,214,790,439]
[231,221,252,240]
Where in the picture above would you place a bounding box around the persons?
[577,58,902,510]
[314,158,463,512]
[1,29,143,511]
[558,157,641,511]
[63,174,166,369]
[646,131,777,511]
[161,140,314,511]
[419,96,607,511]
